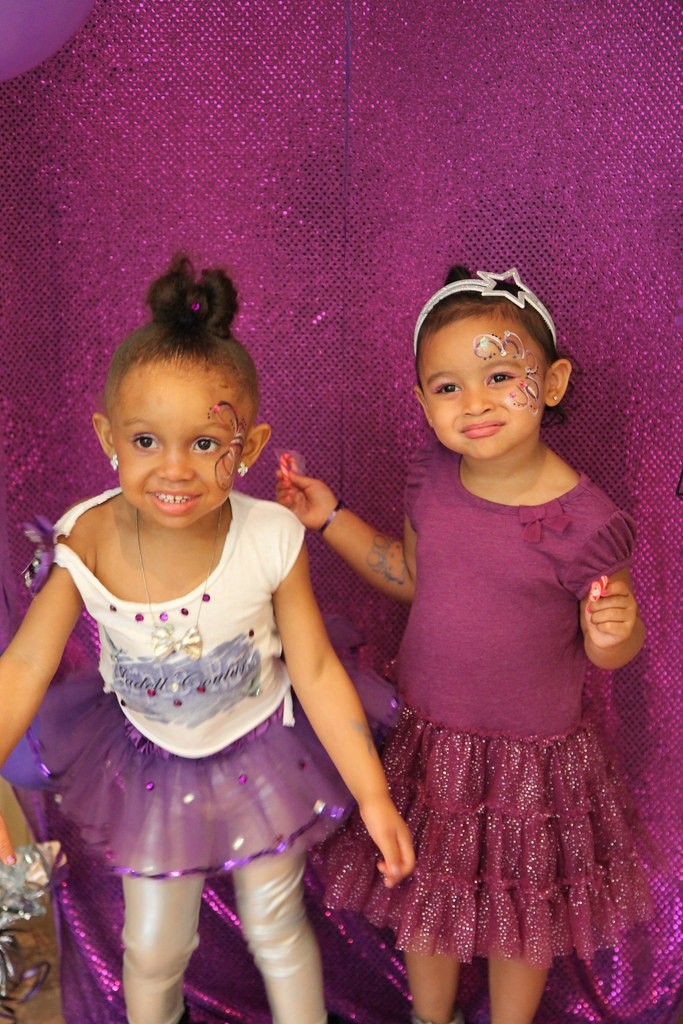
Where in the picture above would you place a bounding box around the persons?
[274,264,648,1024]
[2,260,416,1023]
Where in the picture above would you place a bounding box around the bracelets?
[317,500,343,536]
